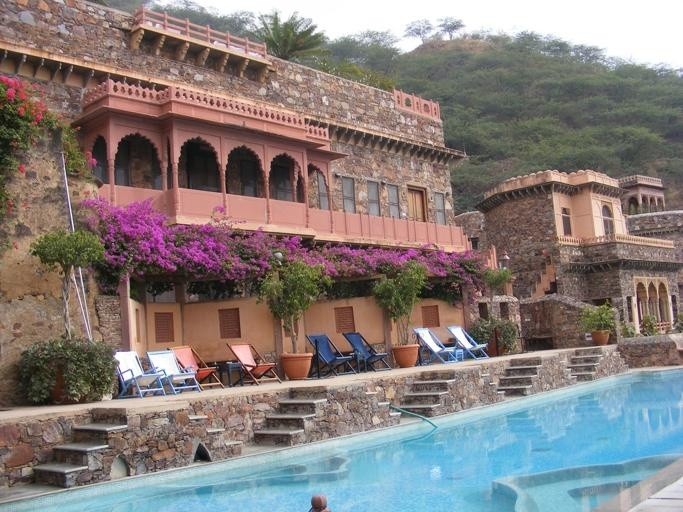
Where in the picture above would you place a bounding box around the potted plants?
[371,259,429,368]
[571,297,619,345]
[254,257,336,379]
[11,224,121,405]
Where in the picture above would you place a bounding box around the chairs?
[226,343,282,386]
[465,267,521,357]
[304,331,392,379]
[114,344,225,399]
[411,326,490,367]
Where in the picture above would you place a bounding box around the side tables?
[207,363,244,389]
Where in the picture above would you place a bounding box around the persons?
[309,494,330,512]
